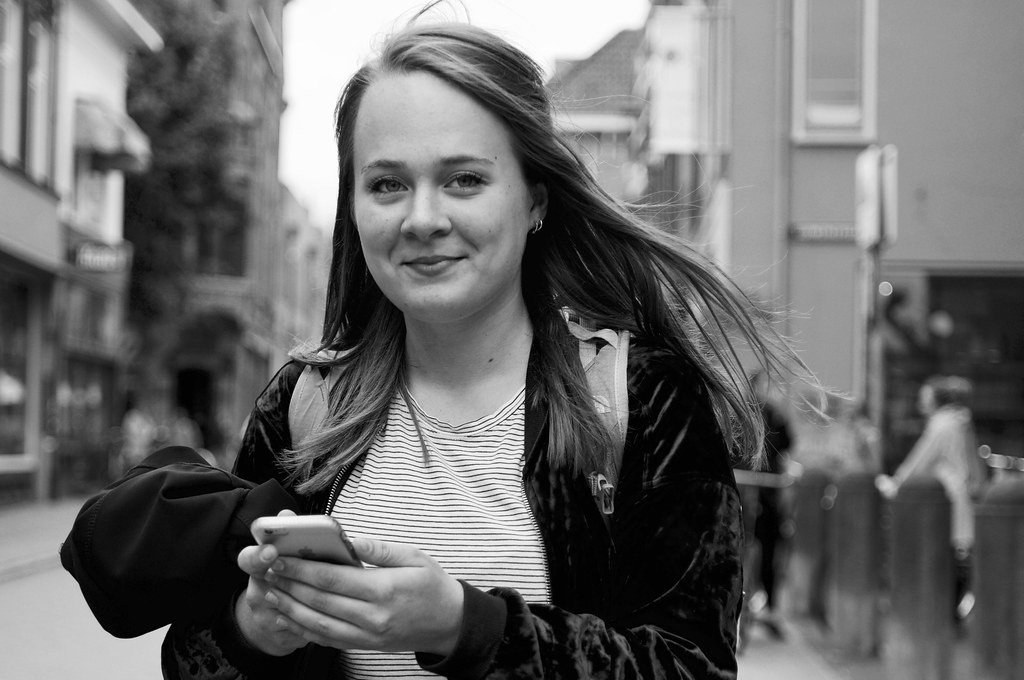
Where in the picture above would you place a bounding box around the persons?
[161,0,852,679]
[874,377,994,623]
[727,371,794,642]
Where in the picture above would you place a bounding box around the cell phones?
[250,515,365,570]
